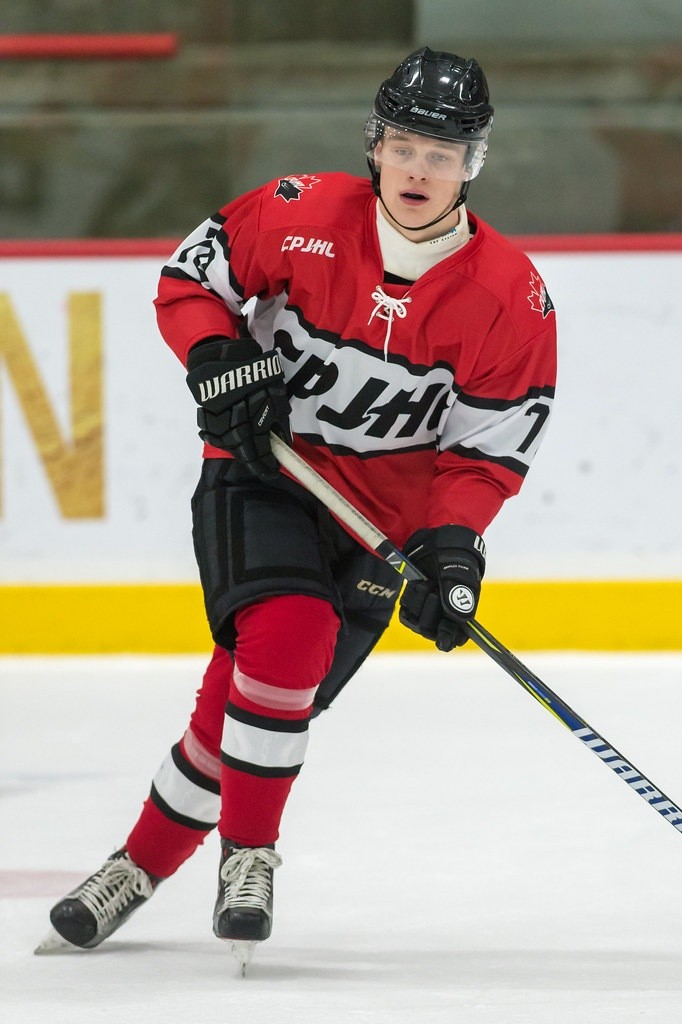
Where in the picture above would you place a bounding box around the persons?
[32,46,558,977]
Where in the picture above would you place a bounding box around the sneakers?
[34,846,166,956]
[212,837,283,980]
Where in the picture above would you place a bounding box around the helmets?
[373,46,495,141]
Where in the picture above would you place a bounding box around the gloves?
[187,337,294,485]
[399,525,486,651]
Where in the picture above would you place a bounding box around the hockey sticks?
[271,431,682,834]
